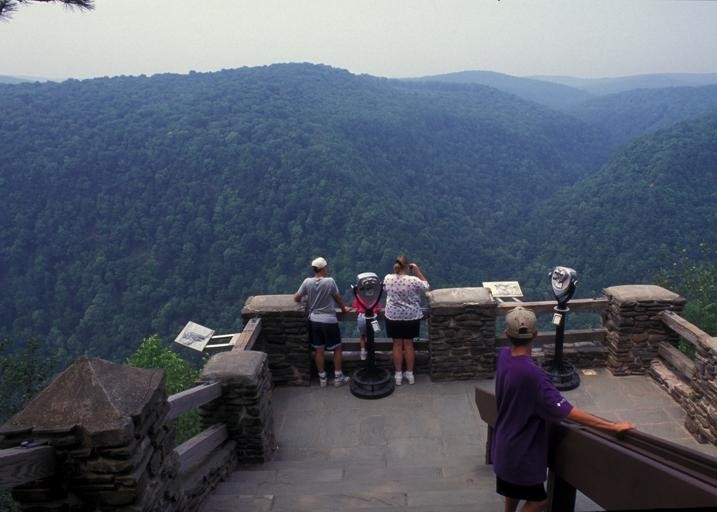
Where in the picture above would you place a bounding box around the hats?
[311,256,327,270]
[505,306,537,339]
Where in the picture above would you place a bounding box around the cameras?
[409,265,413,269]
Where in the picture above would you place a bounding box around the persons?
[492,306,637,512]
[383,253,430,386]
[349,293,382,361]
[295,257,352,388]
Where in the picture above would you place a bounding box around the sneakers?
[320,370,351,388]
[395,375,403,386]
[403,371,415,384]
[360,353,367,361]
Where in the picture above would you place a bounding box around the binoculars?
[409,265,413,269]
